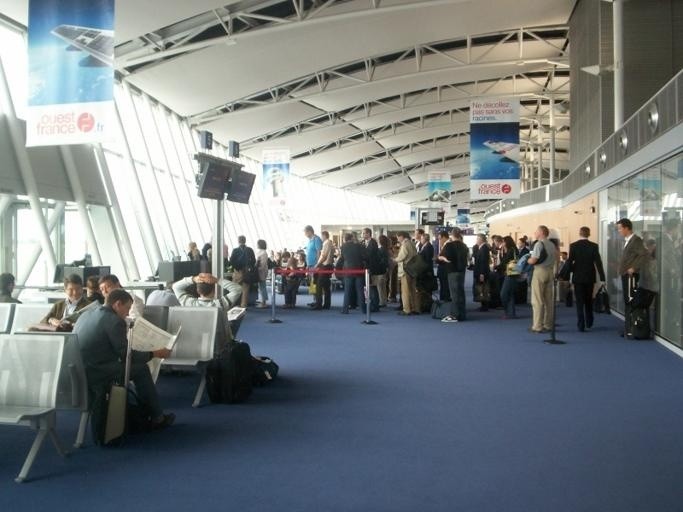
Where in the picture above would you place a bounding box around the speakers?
[228,141,242,158]
[199,130,214,149]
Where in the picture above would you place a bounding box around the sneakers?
[393,305,420,315]
[340,304,386,314]
[255,301,268,307]
[307,302,331,310]
[280,303,295,309]
[441,315,458,322]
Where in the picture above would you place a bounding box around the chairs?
[3,298,238,411]
[1,327,102,483]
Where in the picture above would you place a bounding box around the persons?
[473,223,568,318]
[528,225,556,333]
[86,276,105,305]
[304,223,469,322]
[98,274,144,324]
[0,273,23,304]
[70,289,175,430]
[172,273,245,339]
[146,279,181,307]
[614,219,650,338]
[558,226,606,332]
[28,273,92,331]
[190,233,306,308]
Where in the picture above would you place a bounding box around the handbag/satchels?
[631,287,657,309]
[403,254,428,279]
[595,292,611,315]
[253,356,279,386]
[125,388,151,436]
[535,248,548,264]
[566,290,573,307]
[431,300,455,318]
[506,259,521,276]
[473,284,492,302]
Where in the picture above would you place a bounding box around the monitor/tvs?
[421,211,446,226]
[228,169,256,204]
[200,164,231,204]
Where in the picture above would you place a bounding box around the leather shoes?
[151,413,176,432]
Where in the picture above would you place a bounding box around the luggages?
[624,273,651,340]
[91,322,134,449]
[206,339,254,404]
